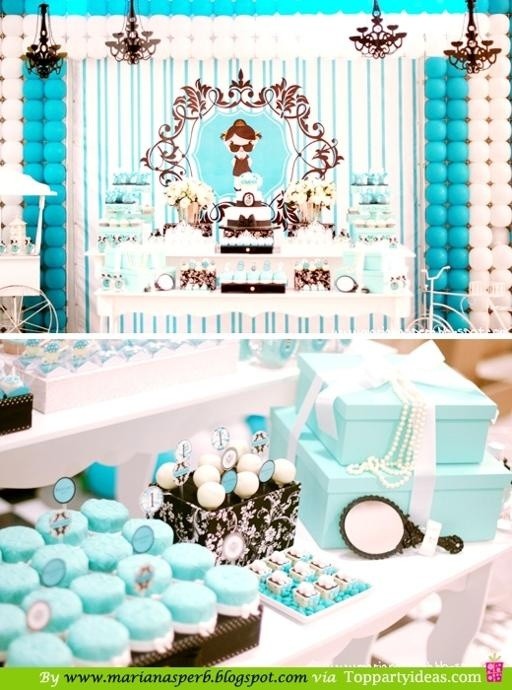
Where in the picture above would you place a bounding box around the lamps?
[443,0,503,78]
[347,1,408,63]
[19,0,68,82]
[104,0,161,69]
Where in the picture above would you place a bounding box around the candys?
[0,427,367,667]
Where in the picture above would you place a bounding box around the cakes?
[218,204,275,246]
